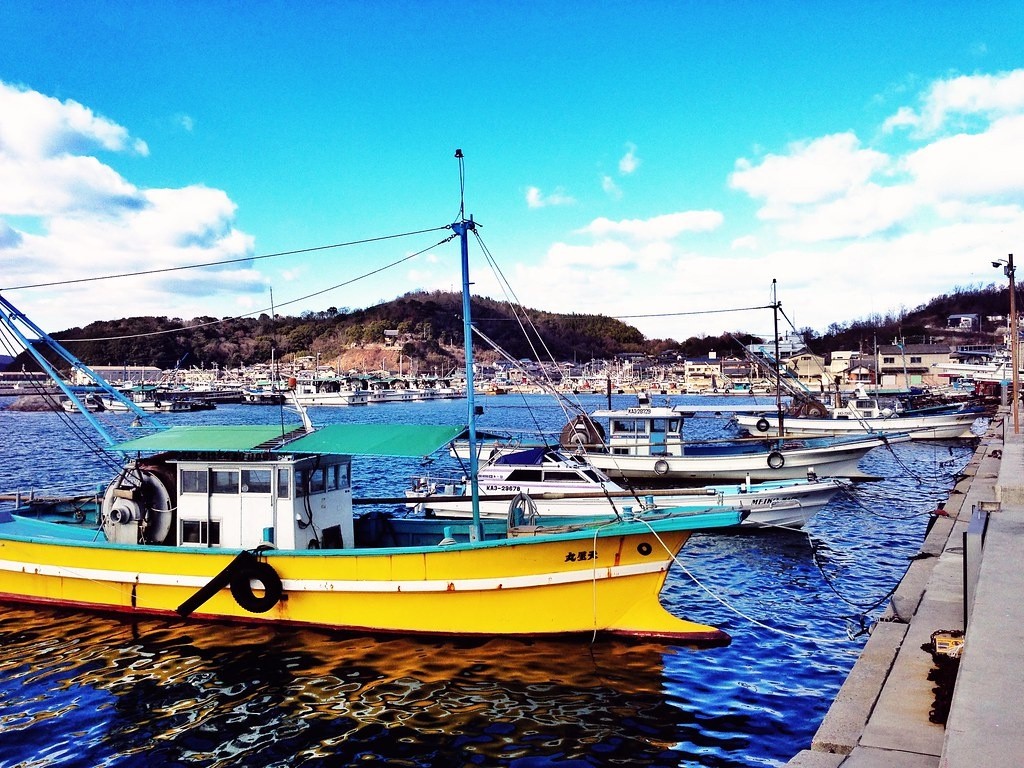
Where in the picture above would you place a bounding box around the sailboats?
[1,144,1002,646]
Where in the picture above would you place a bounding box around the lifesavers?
[155,402,162,408]
[713,388,718,393]
[250,395,254,401]
[749,390,753,394]
[756,418,769,432]
[766,388,770,393]
[654,460,670,476]
[231,557,283,613]
[724,389,729,394]
[768,452,785,470]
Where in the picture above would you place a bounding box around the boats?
[400,446,845,532]
[448,388,913,482]
